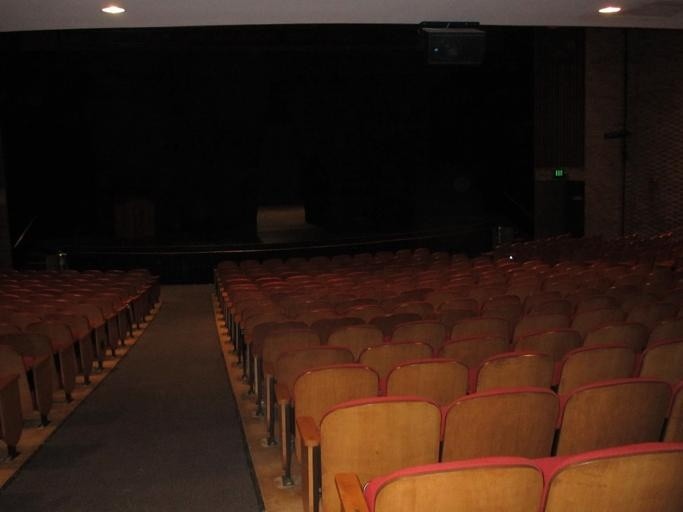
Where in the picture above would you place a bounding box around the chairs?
[0,263,161,492]
